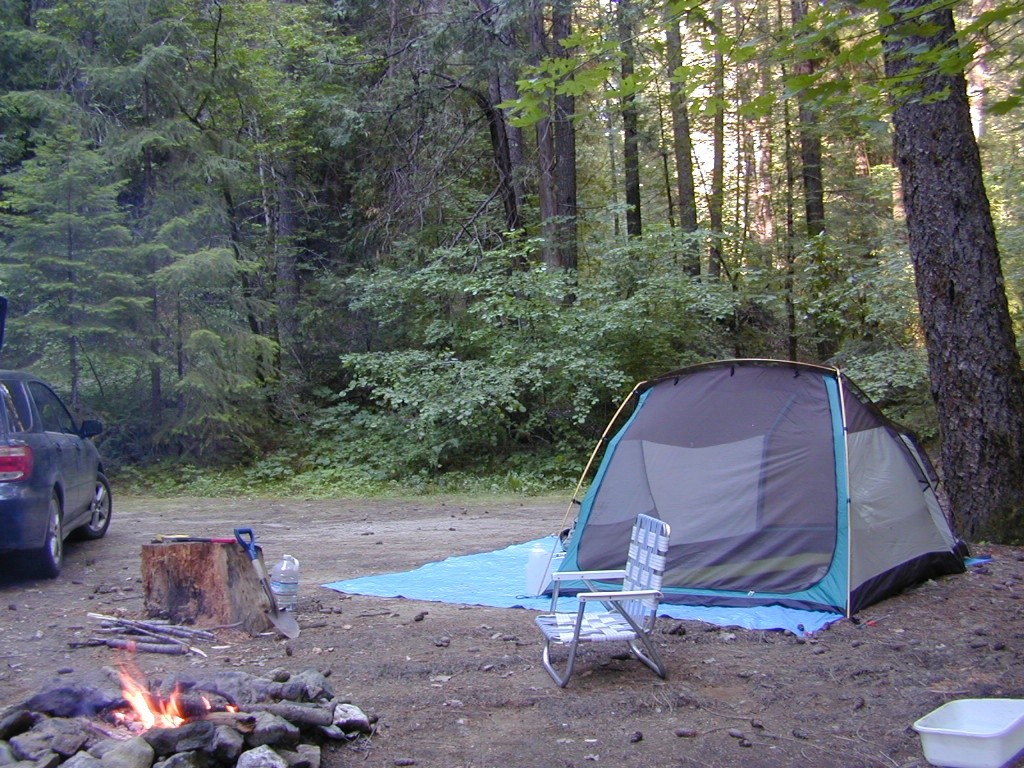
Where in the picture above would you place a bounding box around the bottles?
[269,553,301,611]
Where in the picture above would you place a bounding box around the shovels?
[234,528,302,640]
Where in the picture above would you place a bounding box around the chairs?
[533,512,674,687]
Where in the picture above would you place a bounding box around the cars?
[1,371,113,577]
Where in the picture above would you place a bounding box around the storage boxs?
[911,696,1022,767]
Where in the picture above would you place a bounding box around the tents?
[536,357,969,621]
